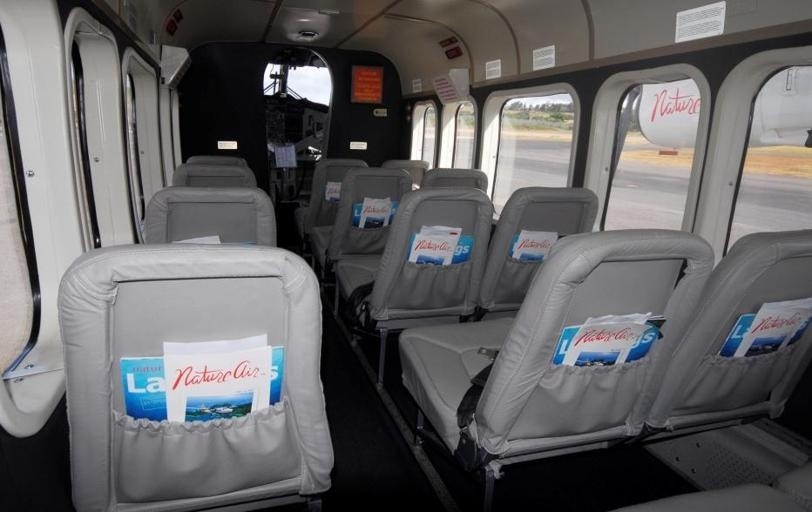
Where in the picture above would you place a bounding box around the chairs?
[55,153,812,512]
